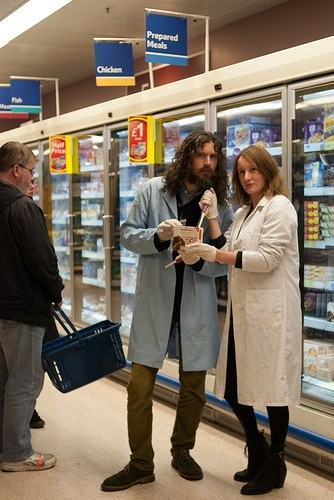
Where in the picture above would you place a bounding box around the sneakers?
[2,452,56,472]
[171,450,203,480]
[100,462,155,491]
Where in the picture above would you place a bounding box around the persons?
[99,129,236,492]
[0,139,64,473]
[15,169,47,428]
[184,143,305,495]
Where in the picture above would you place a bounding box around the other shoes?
[30,410,45,428]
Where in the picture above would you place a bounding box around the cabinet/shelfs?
[0,35,334,477]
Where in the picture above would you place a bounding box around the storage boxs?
[38,319,127,394]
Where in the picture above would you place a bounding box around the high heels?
[234,434,272,482]
[241,452,286,496]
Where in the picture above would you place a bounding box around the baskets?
[40,304,127,394]
